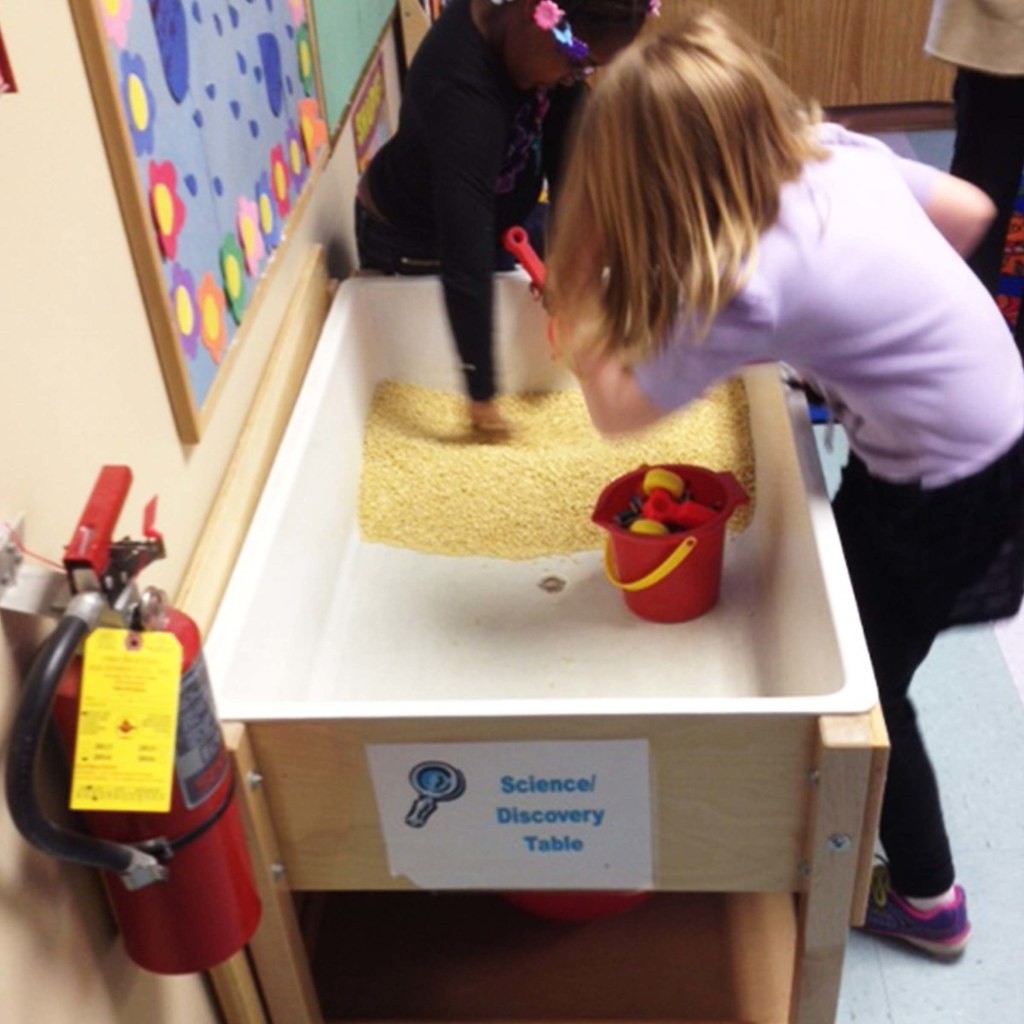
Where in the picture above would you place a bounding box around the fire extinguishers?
[5,466,263,975]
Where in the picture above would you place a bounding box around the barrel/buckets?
[590,462,752,624]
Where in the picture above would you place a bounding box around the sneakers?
[852,866,971,958]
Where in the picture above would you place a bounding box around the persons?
[355,0,652,439]
[530,9,1023,961]
[923,1,1023,289]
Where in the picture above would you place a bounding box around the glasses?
[581,52,600,74]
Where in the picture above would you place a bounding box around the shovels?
[503,226,579,369]
